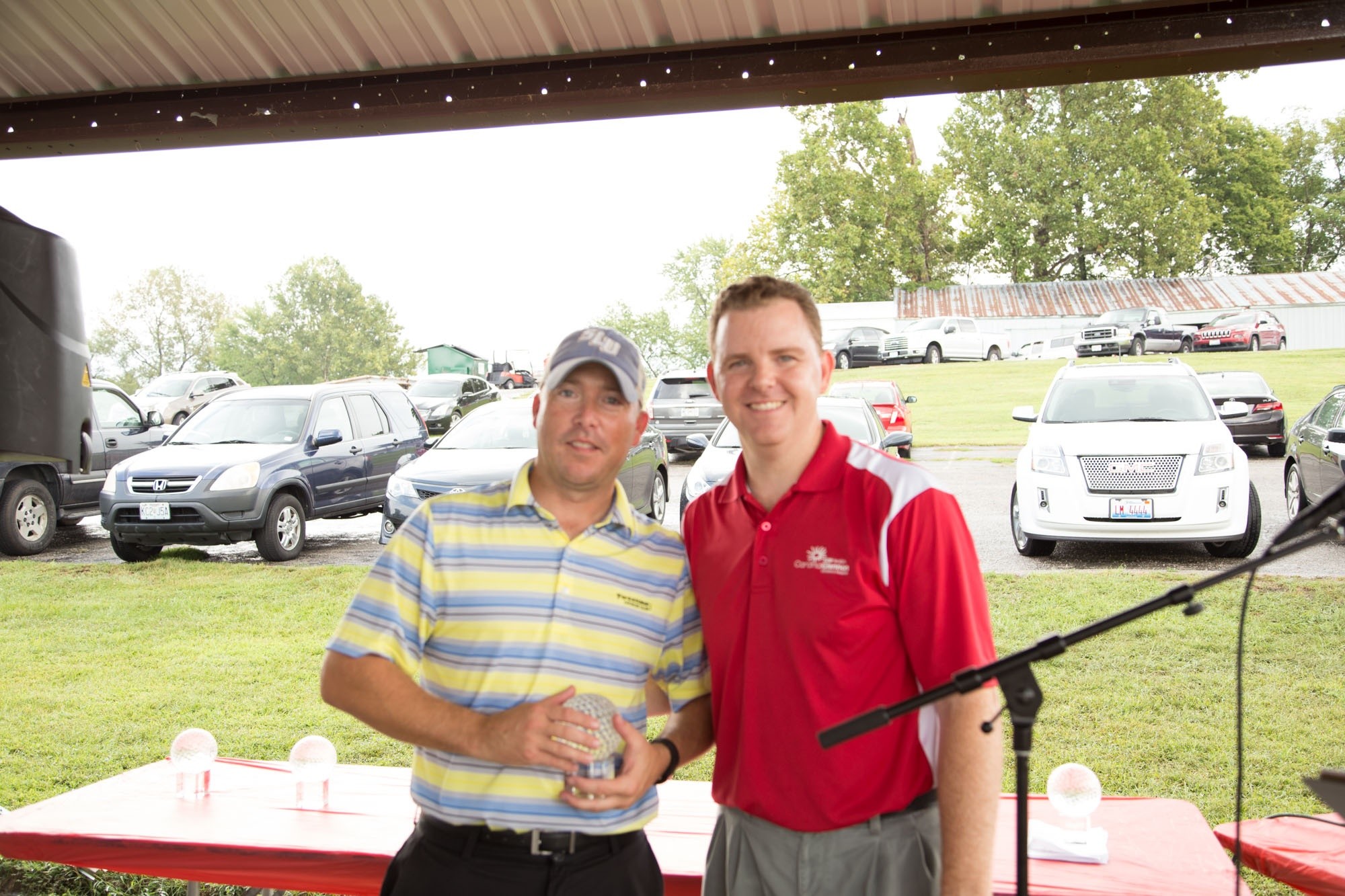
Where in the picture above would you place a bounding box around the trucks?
[0,205,95,556]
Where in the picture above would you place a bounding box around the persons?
[681,274,1007,896]
[319,325,714,896]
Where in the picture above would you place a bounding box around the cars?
[1283,383,1345,530]
[406,373,500,435]
[1196,370,1288,458]
[820,326,922,370]
[679,394,914,525]
[826,380,917,460]
[1193,309,1287,353]
[373,395,669,546]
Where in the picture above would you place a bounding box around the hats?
[545,327,647,405]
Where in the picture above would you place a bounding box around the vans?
[1012,333,1077,361]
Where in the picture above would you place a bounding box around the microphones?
[1271,479,1344,545]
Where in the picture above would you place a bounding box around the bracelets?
[651,738,681,785]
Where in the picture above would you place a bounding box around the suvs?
[99,379,433,564]
[107,369,252,426]
[1010,354,1261,558]
[645,367,726,454]
[58,377,180,524]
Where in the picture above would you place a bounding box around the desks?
[0,756,1257,896]
[1214,812,1345,896]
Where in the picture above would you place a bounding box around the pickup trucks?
[878,316,1011,365]
[500,369,539,390]
[1071,306,1199,357]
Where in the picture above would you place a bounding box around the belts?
[415,807,637,854]
